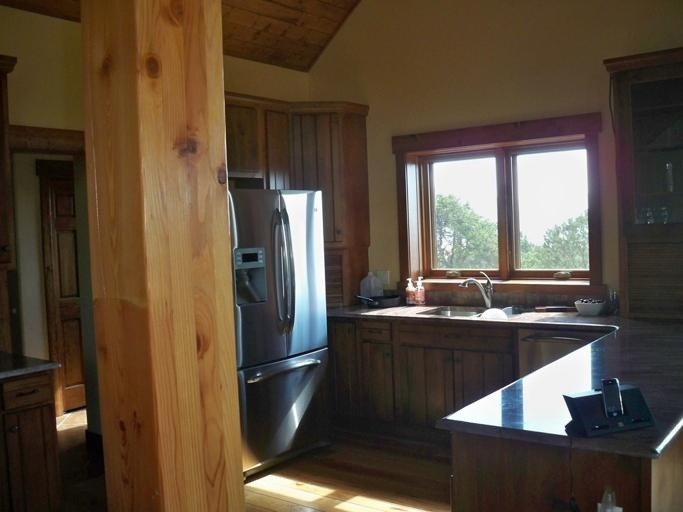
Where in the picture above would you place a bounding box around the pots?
[357,294,402,308]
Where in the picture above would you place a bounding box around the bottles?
[664,159,674,193]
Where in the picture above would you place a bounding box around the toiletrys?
[414,276,426,304]
[405,277,417,306]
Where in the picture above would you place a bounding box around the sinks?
[415,303,486,319]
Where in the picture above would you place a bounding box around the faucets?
[457,270,496,309]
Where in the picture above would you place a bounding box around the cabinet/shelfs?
[0,372,63,511]
[603,46,683,320]
[287,112,371,246]
[396,324,513,431]
[323,319,394,423]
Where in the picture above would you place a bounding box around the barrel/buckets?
[360,272,383,305]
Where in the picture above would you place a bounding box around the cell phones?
[599,377,625,418]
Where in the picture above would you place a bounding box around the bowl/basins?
[574,301,606,317]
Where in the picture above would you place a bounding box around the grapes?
[580,297,603,303]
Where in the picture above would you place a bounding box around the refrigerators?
[228,188,332,479]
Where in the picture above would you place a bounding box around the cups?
[641,207,672,225]
[608,288,616,314]
